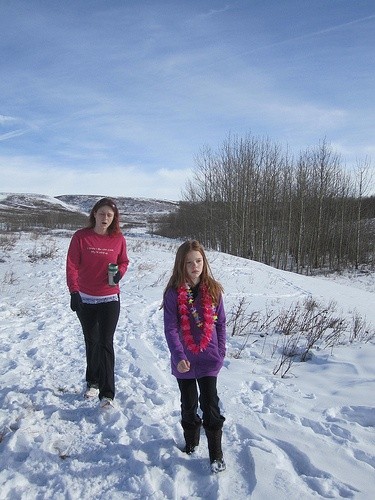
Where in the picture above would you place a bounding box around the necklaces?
[186,283,218,329]
[177,281,215,352]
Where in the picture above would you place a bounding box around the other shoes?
[210,457,226,473]
[83,387,99,399]
[186,445,198,455]
[100,398,114,408]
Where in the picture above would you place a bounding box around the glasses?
[97,200,116,209]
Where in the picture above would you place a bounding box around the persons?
[66,198,129,408]
[160,239,227,473]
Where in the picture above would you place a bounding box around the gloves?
[70,293,84,313]
[113,271,121,284]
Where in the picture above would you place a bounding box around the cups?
[108,264,118,286]
